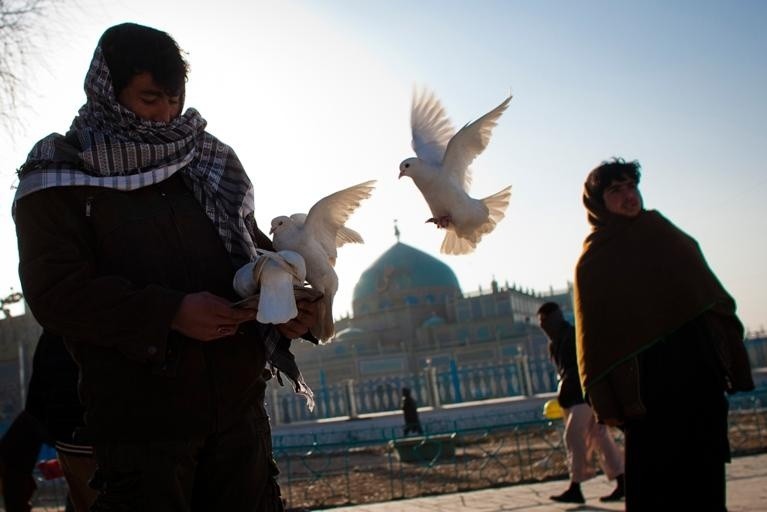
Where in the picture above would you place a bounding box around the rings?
[216,327,234,336]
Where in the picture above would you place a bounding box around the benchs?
[388,432,458,466]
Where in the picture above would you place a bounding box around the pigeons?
[266,179,379,346]
[395,84,517,257]
[233,245,310,330]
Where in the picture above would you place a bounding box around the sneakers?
[550,492,585,504]
[601,487,624,501]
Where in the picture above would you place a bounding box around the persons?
[1,22,318,512]
[537,302,625,503]
[401,388,424,436]
[573,158,755,512]
